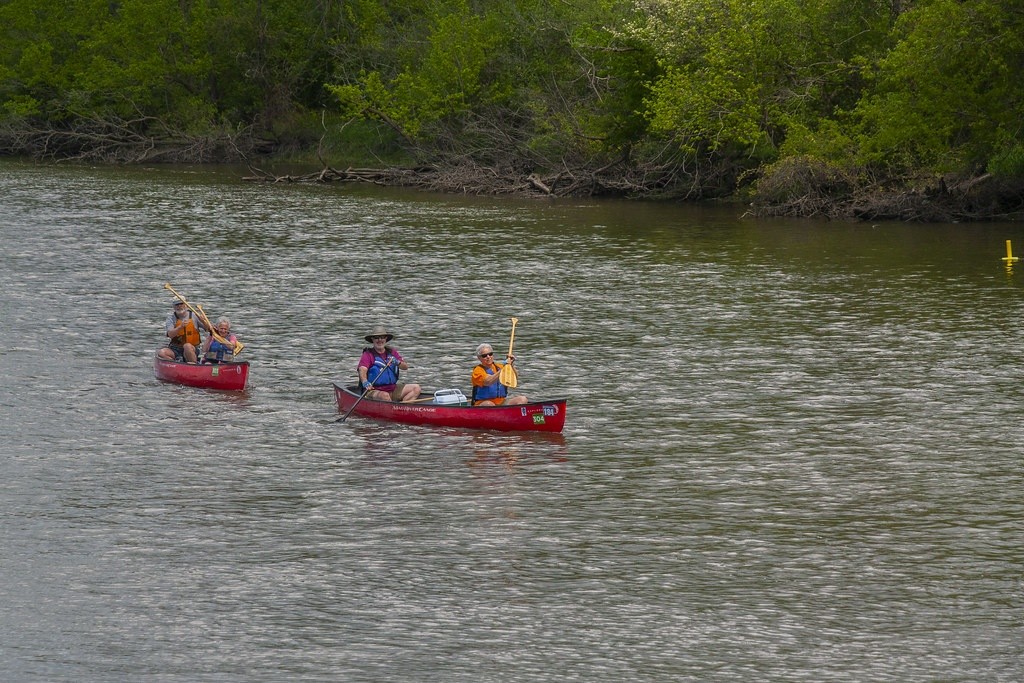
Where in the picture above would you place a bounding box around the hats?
[364,325,393,342]
[173,296,186,305]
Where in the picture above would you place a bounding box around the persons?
[159,296,211,365]
[472,343,527,407]
[201,316,237,365]
[357,328,421,404]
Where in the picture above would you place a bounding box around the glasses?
[479,353,494,357]
[371,335,386,339]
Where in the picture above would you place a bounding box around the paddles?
[330,357,394,423]
[498,318,518,388]
[196,305,232,345]
[164,284,244,356]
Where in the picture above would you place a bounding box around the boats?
[332,381,570,434]
[155,346,249,392]
